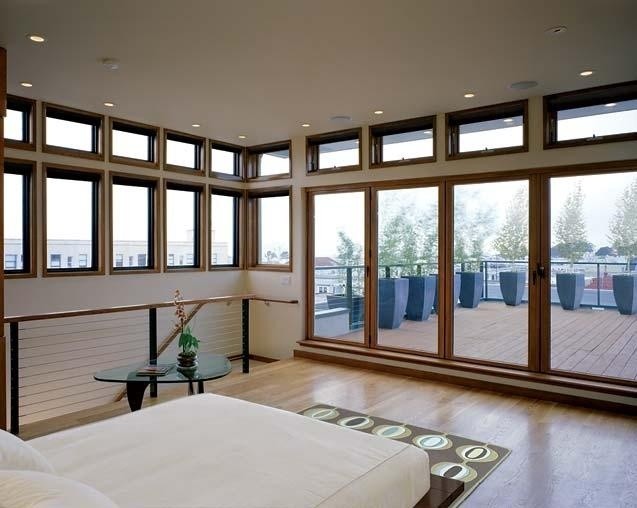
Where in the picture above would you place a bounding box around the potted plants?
[402,273,437,324]
[500,271,526,306]
[556,272,585,310]
[612,273,636,315]
[328,291,364,325]
[377,276,410,329]
[456,271,484,308]
[430,273,462,315]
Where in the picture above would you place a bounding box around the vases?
[176,351,198,371]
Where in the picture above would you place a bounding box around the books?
[135,363,175,377]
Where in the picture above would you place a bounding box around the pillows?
[0,428,120,508]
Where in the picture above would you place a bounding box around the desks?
[94,352,232,413]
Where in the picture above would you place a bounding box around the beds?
[0,391,465,508]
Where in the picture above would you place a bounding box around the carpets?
[294,403,513,508]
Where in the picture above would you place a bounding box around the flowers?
[174,289,201,358]
